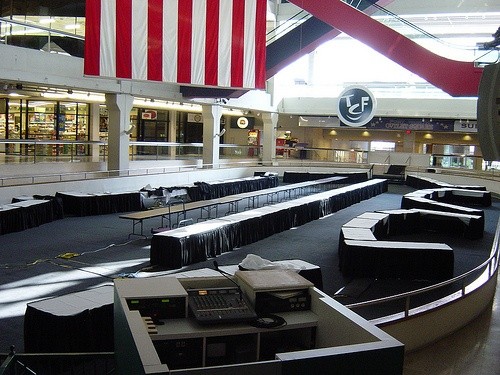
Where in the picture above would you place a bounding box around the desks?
[0,171,492,375]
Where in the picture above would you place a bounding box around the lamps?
[213,128,226,138]
[123,124,136,136]
[274,125,284,130]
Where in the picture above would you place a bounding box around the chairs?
[289,143,308,159]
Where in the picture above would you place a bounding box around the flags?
[83,0,267,91]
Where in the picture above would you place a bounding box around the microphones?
[213,259,243,299]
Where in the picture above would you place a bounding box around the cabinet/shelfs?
[0,112,138,157]
[248,129,261,157]
